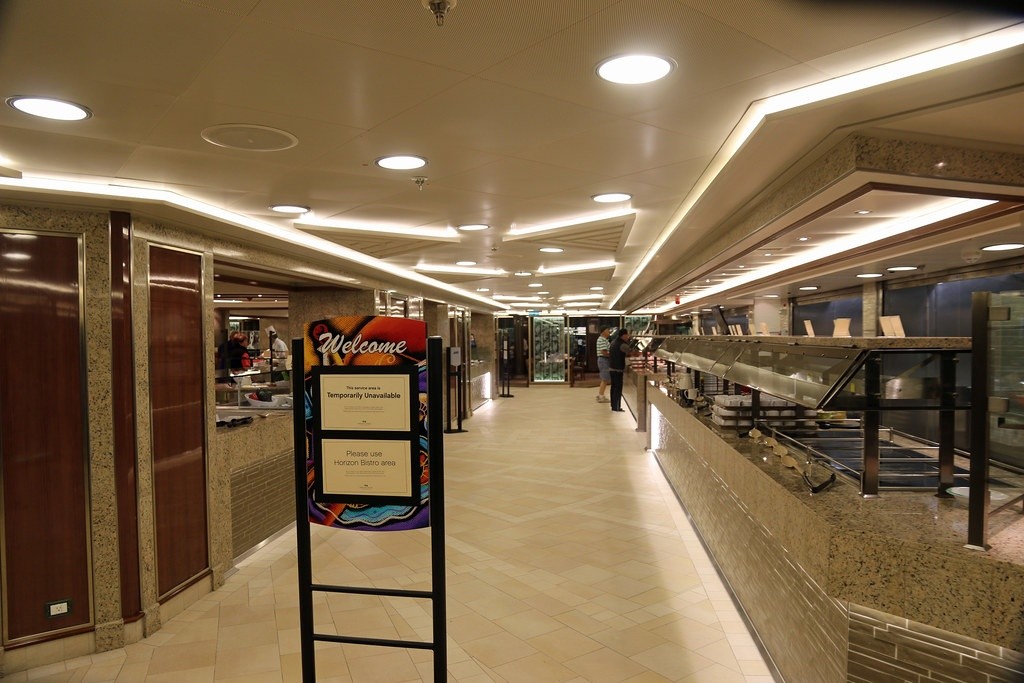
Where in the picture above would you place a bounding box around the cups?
[684,389,699,399]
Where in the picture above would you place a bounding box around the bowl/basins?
[946,487,1009,508]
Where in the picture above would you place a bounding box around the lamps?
[421,0,457,26]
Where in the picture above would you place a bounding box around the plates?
[244,392,277,407]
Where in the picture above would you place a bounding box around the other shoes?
[596,395,610,403]
[612,408,624,412]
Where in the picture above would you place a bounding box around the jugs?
[678,373,693,389]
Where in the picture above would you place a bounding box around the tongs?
[802,471,836,493]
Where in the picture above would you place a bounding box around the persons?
[218,331,250,369]
[252,335,259,349]
[260,326,289,358]
[608,328,632,412]
[596,325,611,403]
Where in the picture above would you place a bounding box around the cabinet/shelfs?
[629,335,973,500]
[215,349,292,410]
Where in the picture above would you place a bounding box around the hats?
[600,324,611,333]
[265,326,277,338]
